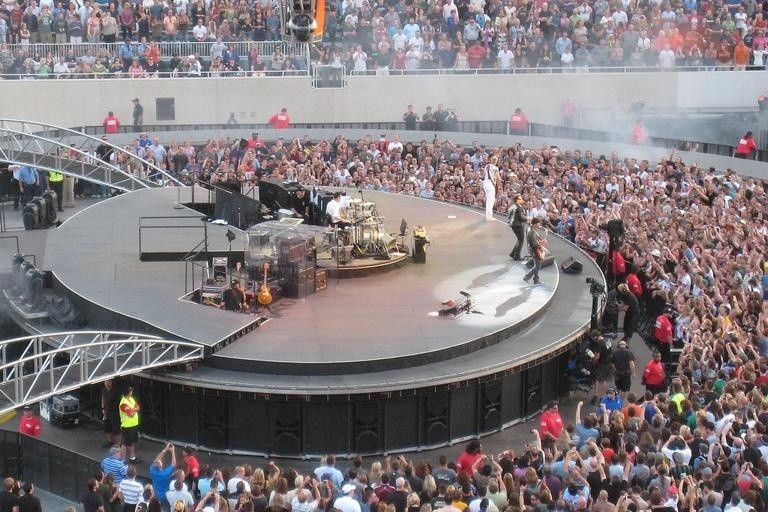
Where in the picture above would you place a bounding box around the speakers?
[561,256,584,274]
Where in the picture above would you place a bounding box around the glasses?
[530,498,537,501]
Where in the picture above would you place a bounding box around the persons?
[482,153,501,222]
[101,378,122,450]
[0,402,516,511]
[0,98,768,211]
[509,194,768,512]
[118,384,143,466]
[291,185,312,225]
[324,192,352,231]
[0,0,768,78]
[215,279,250,312]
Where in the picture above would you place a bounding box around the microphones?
[358,189,362,193]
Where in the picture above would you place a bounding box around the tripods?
[349,229,400,259]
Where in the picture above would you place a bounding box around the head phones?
[623,283,627,292]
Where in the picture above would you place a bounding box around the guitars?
[258,264,272,304]
[495,181,499,199]
[536,229,550,260]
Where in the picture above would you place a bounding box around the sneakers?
[514,256,524,261]
[122,460,129,465]
[509,253,515,260]
[522,279,532,286]
[534,281,545,286]
[486,217,496,221]
[129,457,141,463]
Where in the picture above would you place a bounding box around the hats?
[22,405,32,411]
[341,483,357,495]
[320,471,334,482]
[663,308,674,314]
[296,186,306,191]
[649,248,661,258]
[131,97,139,101]
[108,447,122,455]
[122,385,135,392]
[230,279,240,285]
